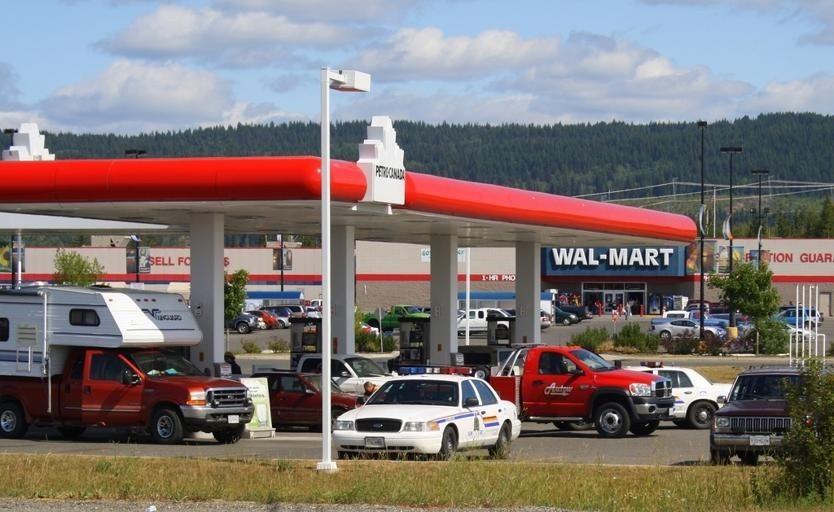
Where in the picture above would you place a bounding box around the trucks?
[0,283,255,445]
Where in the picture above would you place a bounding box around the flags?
[757,224,764,247]
[721,210,733,242]
[699,203,709,237]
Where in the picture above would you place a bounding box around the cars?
[227,298,323,333]
[332,366,524,461]
[247,372,365,427]
[357,320,379,336]
[458,304,593,335]
[646,302,823,349]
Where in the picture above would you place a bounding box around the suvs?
[710,363,825,463]
[625,361,741,428]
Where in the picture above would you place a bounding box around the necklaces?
[623,301,632,320]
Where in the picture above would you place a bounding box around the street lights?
[697,121,710,342]
[750,171,770,268]
[721,146,741,338]
[126,148,147,281]
[318,63,371,472]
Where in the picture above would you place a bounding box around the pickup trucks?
[257,351,399,402]
[364,304,430,330]
[483,341,674,441]
